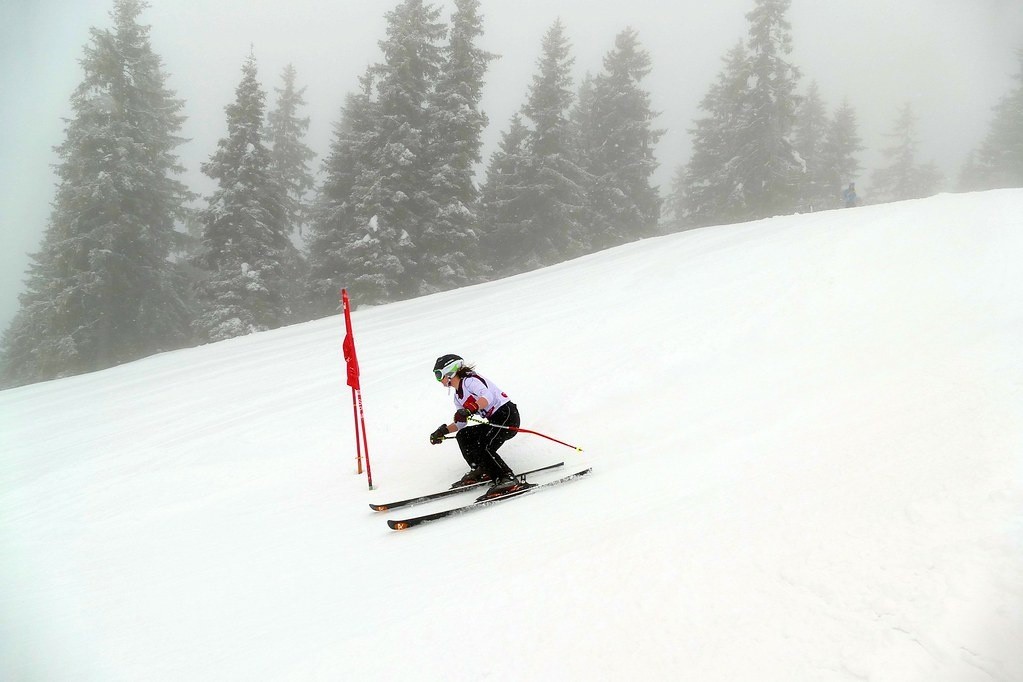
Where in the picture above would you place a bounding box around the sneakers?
[487,473,519,495]
[451,466,492,488]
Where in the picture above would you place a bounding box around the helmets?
[433,354,464,379]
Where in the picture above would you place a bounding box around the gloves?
[454,408,471,423]
[430,424,449,445]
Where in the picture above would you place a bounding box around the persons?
[430,354,520,498]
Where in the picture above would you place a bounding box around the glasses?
[434,369,444,382]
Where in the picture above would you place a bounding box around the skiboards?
[367,459,596,530]
